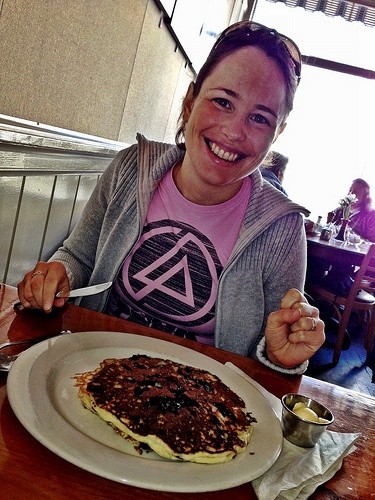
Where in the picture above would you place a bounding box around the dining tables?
[0,282,375,500]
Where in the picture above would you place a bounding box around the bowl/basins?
[279,393,335,449]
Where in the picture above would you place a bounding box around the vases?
[336,220,351,242]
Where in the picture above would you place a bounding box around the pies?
[78,356,258,464]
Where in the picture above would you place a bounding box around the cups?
[320,228,332,240]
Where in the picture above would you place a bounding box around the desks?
[306,234,375,265]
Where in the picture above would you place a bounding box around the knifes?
[12,281,114,308]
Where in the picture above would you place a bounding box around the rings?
[311,317,316,331]
[32,271,44,279]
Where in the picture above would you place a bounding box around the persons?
[258,151,375,275]
[16,21,324,372]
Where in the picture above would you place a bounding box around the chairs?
[312,244,375,364]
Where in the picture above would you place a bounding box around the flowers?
[340,194,359,219]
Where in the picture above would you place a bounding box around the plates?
[7,330,285,493]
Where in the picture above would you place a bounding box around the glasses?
[214,20,301,86]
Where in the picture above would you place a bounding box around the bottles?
[317,216,324,225]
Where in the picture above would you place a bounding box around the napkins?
[226,362,360,500]
